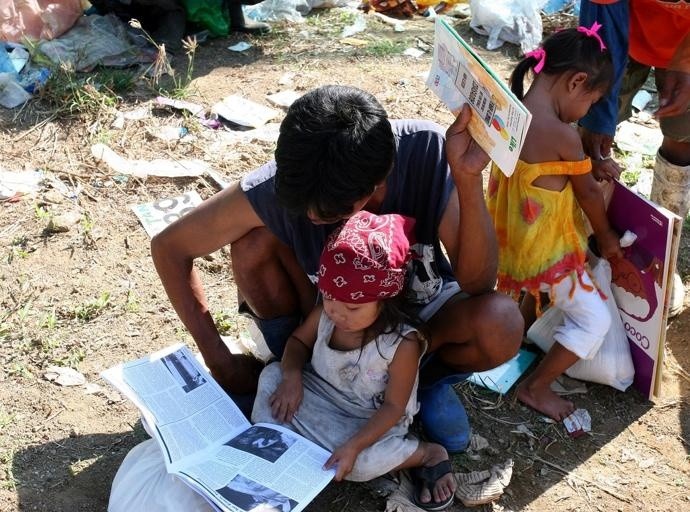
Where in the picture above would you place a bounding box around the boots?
[650,148,690,318]
[417,347,474,453]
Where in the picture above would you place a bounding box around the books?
[100,343,338,512]
[423,15,534,177]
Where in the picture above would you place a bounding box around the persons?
[486,20,625,422]
[251,211,458,512]
[577,0,690,318]
[151,86,524,454]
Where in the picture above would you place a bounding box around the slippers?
[412,460,457,511]
